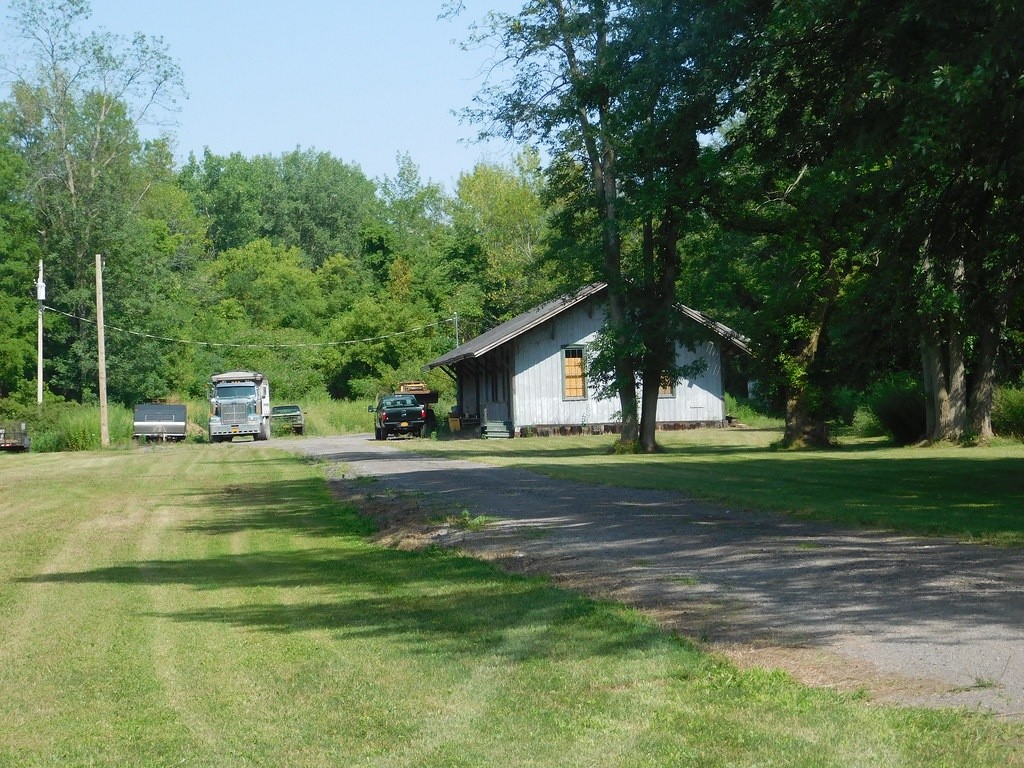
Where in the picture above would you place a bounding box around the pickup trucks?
[368,395,426,439]
[270,405,308,434]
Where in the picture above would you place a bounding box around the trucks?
[209,372,271,443]
